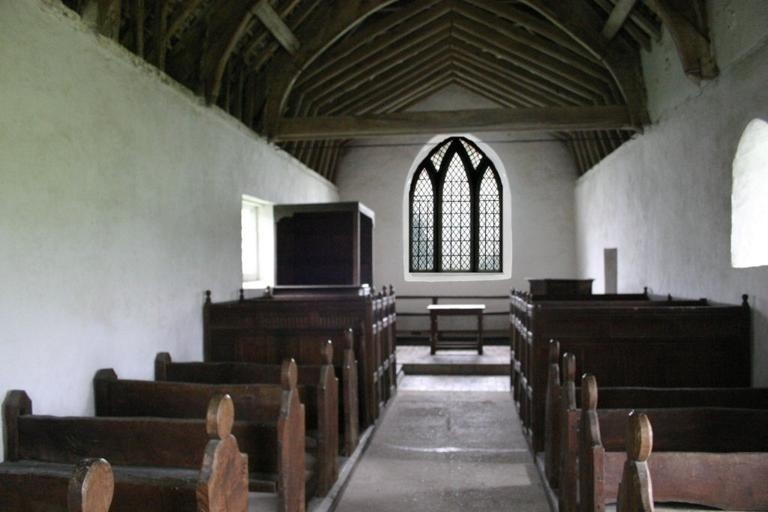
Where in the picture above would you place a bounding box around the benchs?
[546,337,766,510]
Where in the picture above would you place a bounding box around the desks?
[426,305,485,354]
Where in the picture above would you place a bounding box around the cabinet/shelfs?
[509,287,752,462]
[203,283,396,428]
